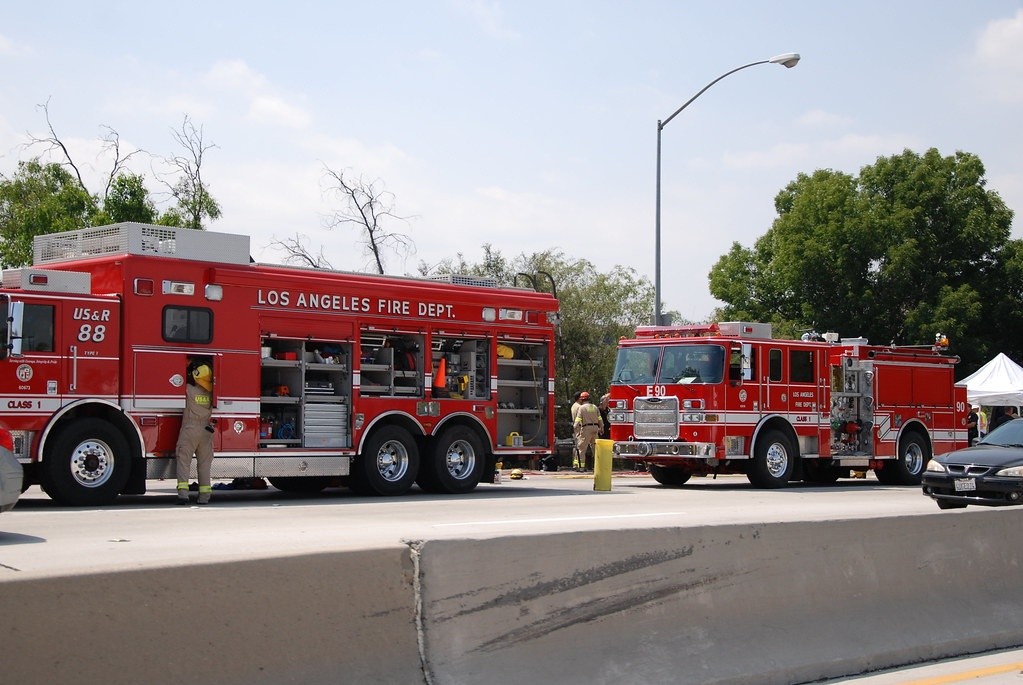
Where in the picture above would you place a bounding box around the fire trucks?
[0,219,564,512]
[606,320,971,489]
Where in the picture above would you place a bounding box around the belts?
[583,423,599,426]
[579,424,583,427]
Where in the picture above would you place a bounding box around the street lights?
[655,53,801,328]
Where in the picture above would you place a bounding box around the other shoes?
[196,499,206,504]
[580,468,587,471]
[176,496,188,505]
[573,465,579,469]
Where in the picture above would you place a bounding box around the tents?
[954,352,1023,437]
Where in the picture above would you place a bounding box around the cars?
[920,417,1023,510]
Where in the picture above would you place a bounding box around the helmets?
[579,392,589,399]
[192,365,214,392]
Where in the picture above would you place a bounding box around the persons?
[967,403,987,447]
[175,365,215,504]
[997,406,1015,427]
[571,391,611,472]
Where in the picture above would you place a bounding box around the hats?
[971,405,979,409]
[574,391,581,401]
